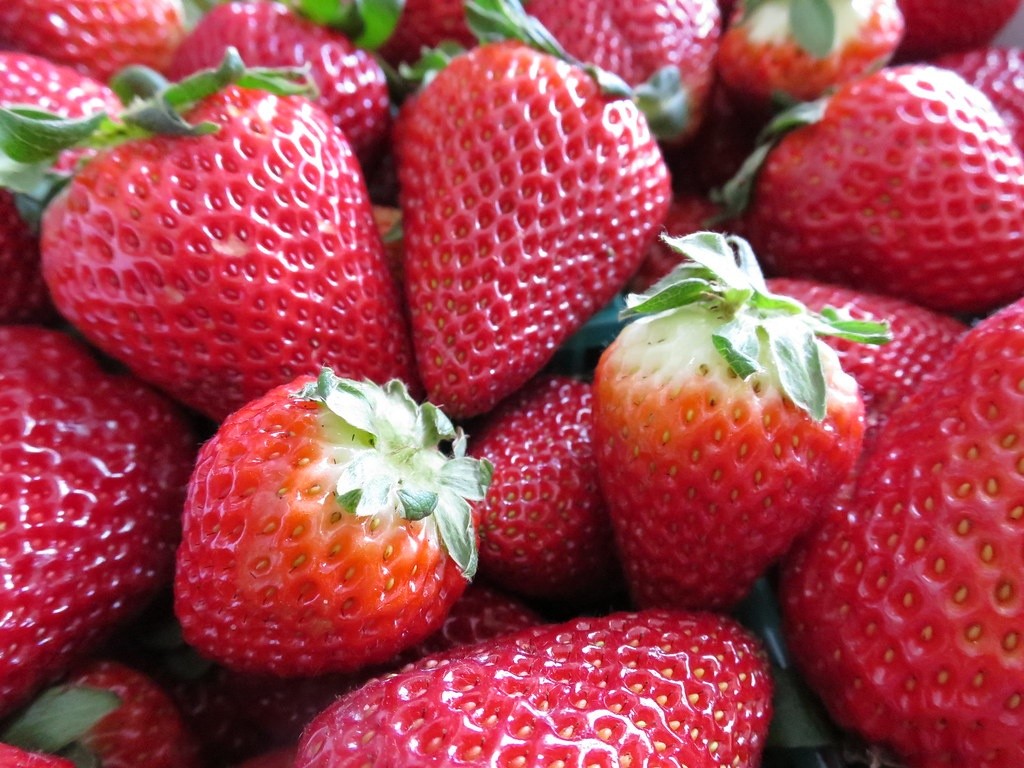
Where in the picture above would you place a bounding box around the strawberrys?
[1,1,1024,768]
